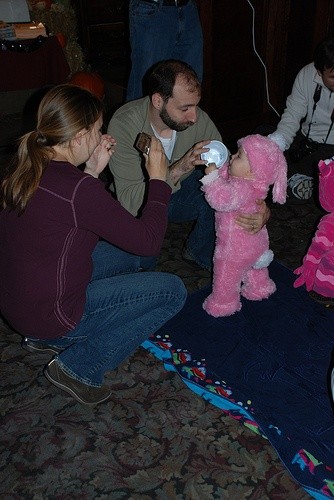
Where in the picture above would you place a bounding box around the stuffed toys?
[294,158,334,298]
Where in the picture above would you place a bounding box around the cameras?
[135,133,152,156]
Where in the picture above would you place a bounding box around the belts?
[141,0,188,6]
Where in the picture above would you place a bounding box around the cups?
[201,141,228,168]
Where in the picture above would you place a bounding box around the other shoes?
[22,336,63,357]
[178,258,214,278]
[44,359,113,405]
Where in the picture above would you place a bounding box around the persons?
[0,85,186,405]
[199,134,287,317]
[107,60,223,274]
[263,37,334,211]
[127,0,203,103]
